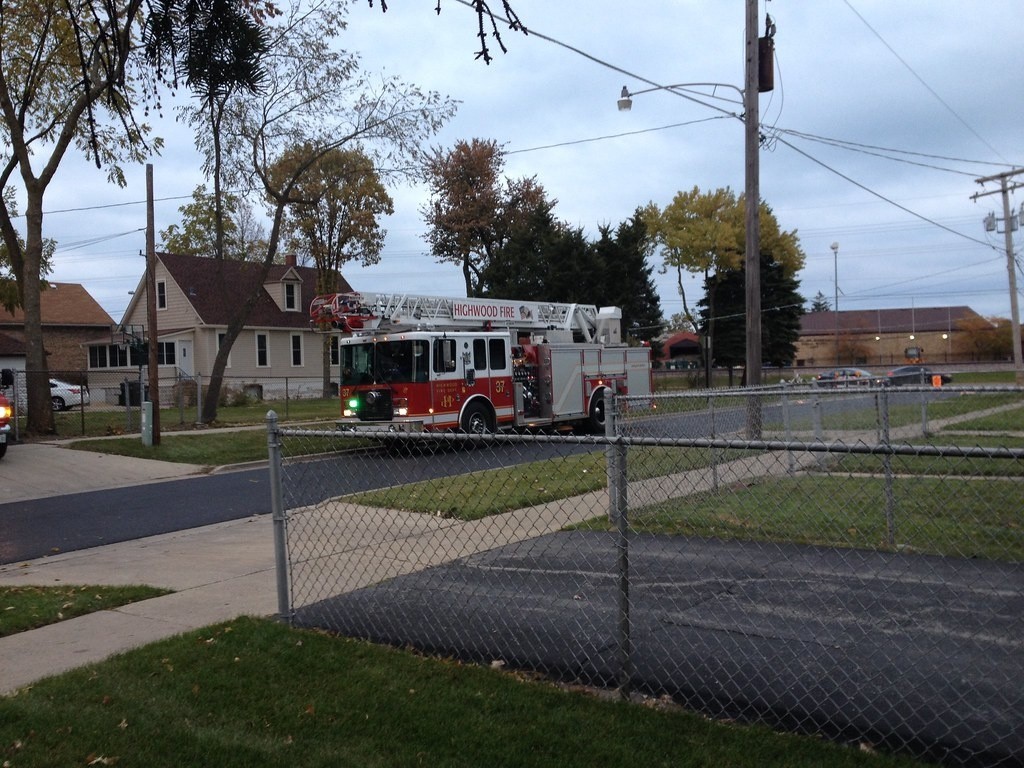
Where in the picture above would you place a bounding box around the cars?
[885,366,954,386]
[46,378,90,411]
[817,368,889,389]
[0,368,15,458]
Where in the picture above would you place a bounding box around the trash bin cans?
[329,382,339,398]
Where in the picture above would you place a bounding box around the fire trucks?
[307,291,656,448]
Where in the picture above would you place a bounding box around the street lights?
[830,241,840,364]
[910,335,916,362]
[943,334,951,363]
[614,82,763,441]
[875,337,882,364]
